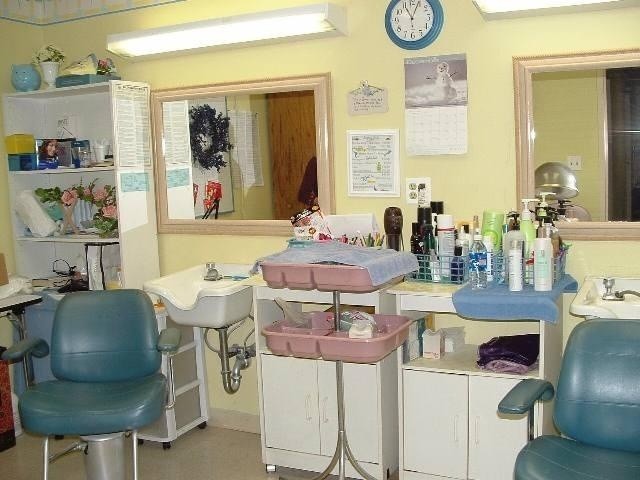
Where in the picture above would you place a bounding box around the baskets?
[409,253,564,286]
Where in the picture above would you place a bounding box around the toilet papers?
[12,189,60,237]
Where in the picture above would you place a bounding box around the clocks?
[382,0,445,51]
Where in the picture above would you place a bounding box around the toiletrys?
[535,192,580,223]
[501,198,561,293]
[480,210,505,253]
[405,190,562,293]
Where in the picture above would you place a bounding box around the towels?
[451,272,580,327]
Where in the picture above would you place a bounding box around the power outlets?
[564,154,583,172]
[405,176,432,205]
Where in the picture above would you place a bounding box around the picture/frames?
[346,128,401,198]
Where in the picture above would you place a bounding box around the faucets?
[601,276,640,300]
[203,262,224,281]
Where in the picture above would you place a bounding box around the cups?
[92,144,110,162]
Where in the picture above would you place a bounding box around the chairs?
[497,316,640,479]
[1,287,183,480]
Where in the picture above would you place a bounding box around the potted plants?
[27,41,68,89]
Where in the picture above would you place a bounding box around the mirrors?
[511,47,638,242]
[149,71,337,241]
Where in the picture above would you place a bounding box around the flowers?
[31,175,120,238]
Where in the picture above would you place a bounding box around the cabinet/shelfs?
[253,274,406,480]
[1,79,160,442]
[137,311,210,451]
[385,279,566,480]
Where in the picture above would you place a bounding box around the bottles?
[70,140,95,165]
[532,237,554,292]
[410,199,465,281]
[468,234,487,289]
[508,239,524,292]
[76,150,92,168]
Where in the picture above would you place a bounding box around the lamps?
[105,2,349,68]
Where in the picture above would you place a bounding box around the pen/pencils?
[333,231,386,247]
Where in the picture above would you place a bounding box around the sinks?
[142,261,262,328]
[569,276,640,320]
